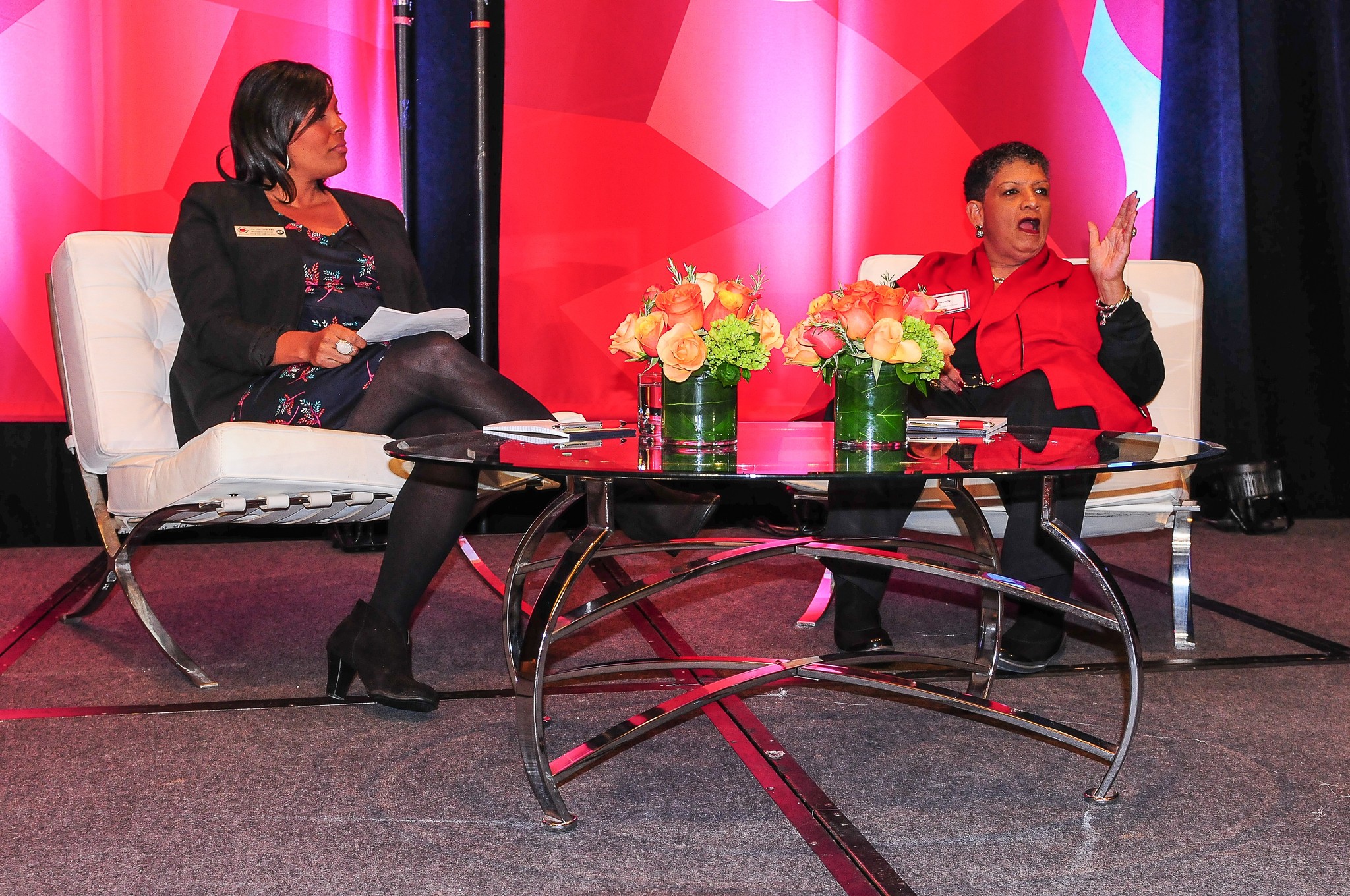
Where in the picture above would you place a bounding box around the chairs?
[0,228,585,694]
[792,255,1209,650]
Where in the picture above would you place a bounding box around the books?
[907,415,1008,434]
[906,425,1007,438]
[483,419,637,439]
[483,431,637,444]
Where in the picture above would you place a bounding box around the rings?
[1121,227,1137,238]
[335,339,353,356]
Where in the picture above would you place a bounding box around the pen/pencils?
[908,437,996,444]
[907,418,996,429]
[552,419,628,430]
[552,435,627,449]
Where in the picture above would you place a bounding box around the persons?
[169,57,724,712]
[818,140,1166,674]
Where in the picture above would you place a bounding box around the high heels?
[324,599,441,712]
[614,492,721,558]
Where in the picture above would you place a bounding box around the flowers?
[607,254,786,390]
[782,272,957,403]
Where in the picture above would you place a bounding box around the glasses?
[931,373,998,391]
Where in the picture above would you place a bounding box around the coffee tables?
[375,419,1235,830]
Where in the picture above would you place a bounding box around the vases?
[658,357,738,449]
[832,367,907,452]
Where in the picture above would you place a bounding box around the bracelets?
[1096,284,1132,327]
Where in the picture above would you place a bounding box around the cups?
[637,435,663,470]
[637,374,663,435]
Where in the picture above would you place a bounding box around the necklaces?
[993,274,1005,284]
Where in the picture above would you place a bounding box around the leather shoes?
[833,595,895,657]
[997,624,1068,674]
